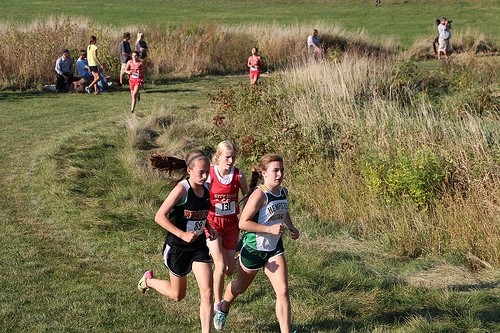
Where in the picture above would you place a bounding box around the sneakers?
[213,300,229,330]
[138,271,153,292]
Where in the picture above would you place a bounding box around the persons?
[431,18,453,61]
[138,149,218,333]
[119,32,132,85]
[307,29,322,53]
[213,154,300,333]
[247,47,262,85]
[55,49,112,93]
[85,36,104,95]
[124,51,144,113]
[134,32,148,65]
[204,141,249,315]
